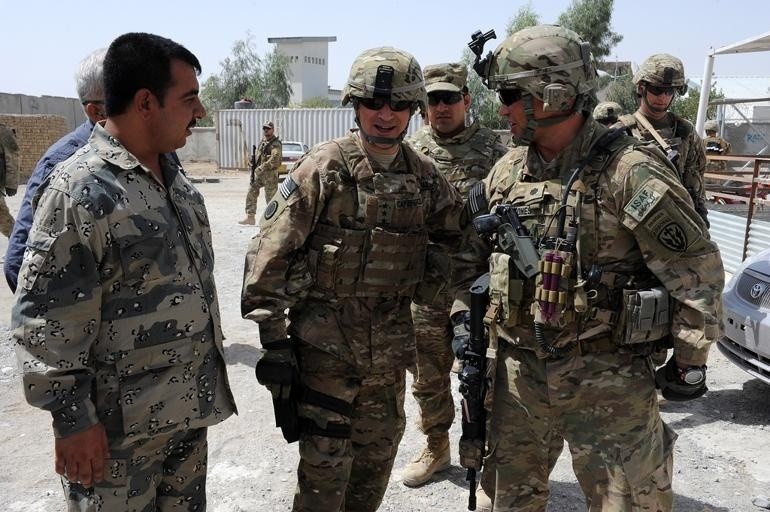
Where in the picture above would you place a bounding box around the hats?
[262,120,274,128]
[424,63,469,91]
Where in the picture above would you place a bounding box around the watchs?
[675,364,707,385]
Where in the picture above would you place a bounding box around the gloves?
[651,357,710,404]
[256,341,304,399]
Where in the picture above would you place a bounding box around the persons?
[240,45,465,512]
[3,48,110,296]
[701,117,730,185]
[233,95,255,109]
[238,121,283,226]
[400,61,509,488]
[607,48,710,367]
[591,101,625,129]
[1,118,20,240]
[403,105,465,387]
[8,31,239,512]
[447,23,726,510]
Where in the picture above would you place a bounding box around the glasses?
[352,98,413,110]
[498,90,524,106]
[640,81,677,96]
[427,94,464,104]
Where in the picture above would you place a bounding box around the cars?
[277,141,309,177]
[720,249,770,386]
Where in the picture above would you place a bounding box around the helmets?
[594,102,624,120]
[339,46,431,127]
[632,54,685,88]
[483,26,601,112]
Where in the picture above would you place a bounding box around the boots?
[238,215,257,226]
[401,433,455,492]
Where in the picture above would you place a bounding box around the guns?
[458,273,491,511]
[248,142,258,186]
[471,196,542,278]
